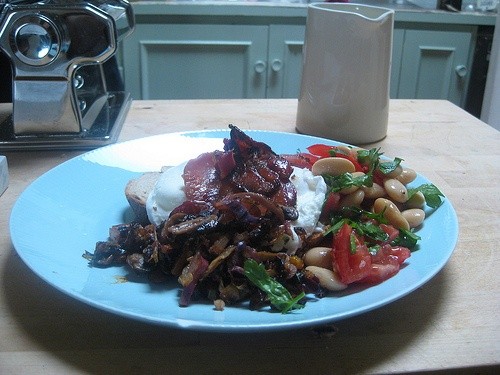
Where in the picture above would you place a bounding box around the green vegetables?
[244,145,446,316]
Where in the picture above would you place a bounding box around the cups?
[294,3,396,144]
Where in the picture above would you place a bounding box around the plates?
[10,130,460,332]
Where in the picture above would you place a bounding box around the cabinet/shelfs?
[114,1,497,110]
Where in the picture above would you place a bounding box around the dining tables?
[0,99,500,375]
